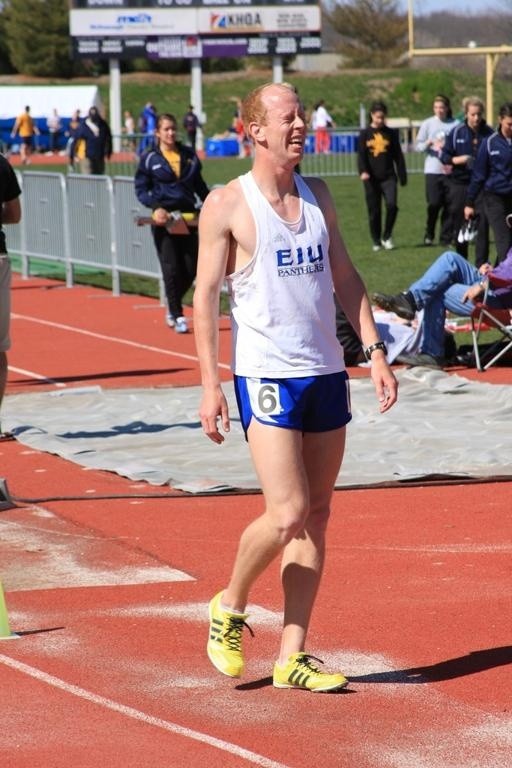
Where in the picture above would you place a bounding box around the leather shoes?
[370,289,416,320]
[394,350,444,371]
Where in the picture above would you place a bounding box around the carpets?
[0,365,512,495]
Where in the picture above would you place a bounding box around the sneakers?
[164,307,188,335]
[207,588,254,678]
[268,651,353,694]
[371,234,453,253]
[454,214,483,243]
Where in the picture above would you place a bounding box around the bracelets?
[365,341,389,360]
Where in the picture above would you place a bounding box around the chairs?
[444,271,512,373]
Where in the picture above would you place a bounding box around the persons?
[1,155,22,410]
[463,102,512,269]
[190,81,401,695]
[412,93,460,246]
[235,100,336,159]
[356,98,409,252]
[370,211,512,368]
[10,106,112,175]
[124,102,202,159]
[133,112,209,337]
[437,96,495,256]
